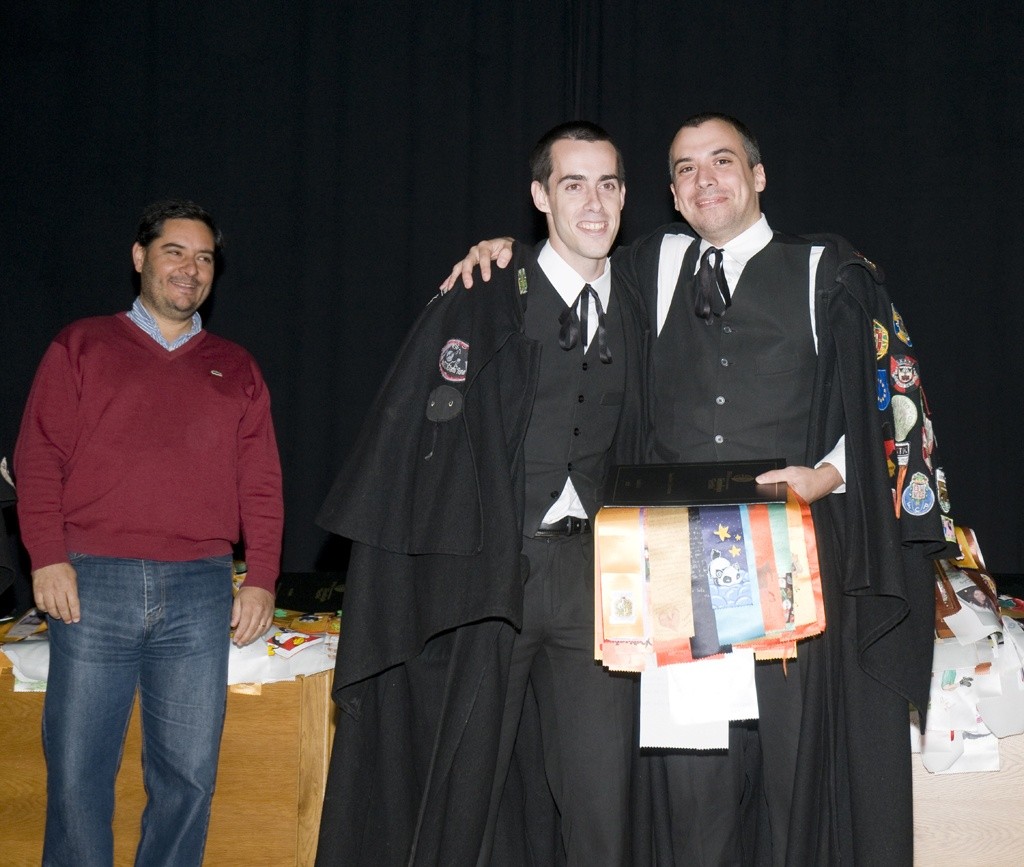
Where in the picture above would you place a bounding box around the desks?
[0,615,1024,867]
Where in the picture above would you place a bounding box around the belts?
[538,517,590,536]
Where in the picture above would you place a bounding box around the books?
[602,458,786,509]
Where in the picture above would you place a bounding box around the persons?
[314,122,650,867]
[13,202,283,867]
[439,109,962,867]
[970,586,993,610]
